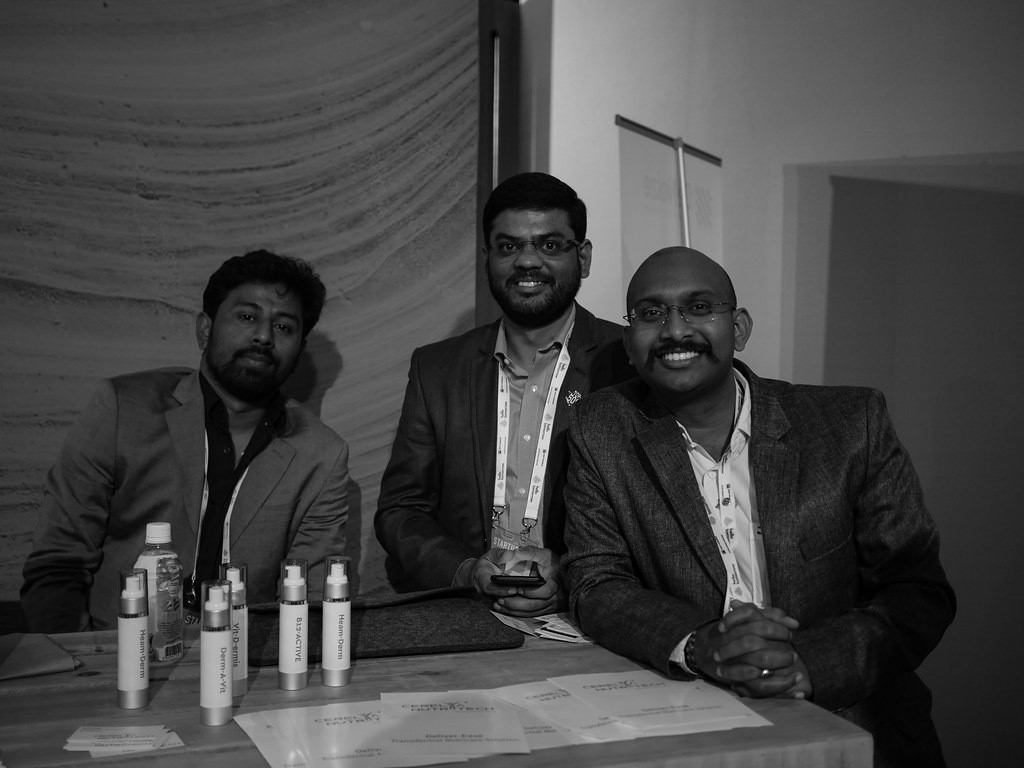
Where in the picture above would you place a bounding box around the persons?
[374,170,635,619]
[564,246,957,768]
[20,250,351,633]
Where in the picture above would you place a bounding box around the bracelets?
[683,629,700,671]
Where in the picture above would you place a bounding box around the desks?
[0,613,874,768]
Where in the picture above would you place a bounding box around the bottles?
[132,521,184,666]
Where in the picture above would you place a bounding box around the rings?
[759,666,772,678]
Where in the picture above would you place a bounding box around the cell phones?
[490,574,545,590]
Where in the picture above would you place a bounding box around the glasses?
[623,302,733,324]
[484,240,581,260]
